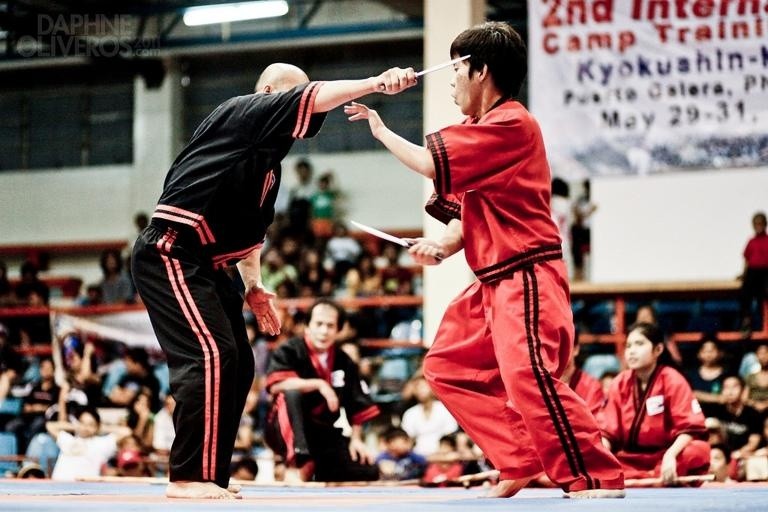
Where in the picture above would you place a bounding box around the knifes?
[347,218,445,261]
[377,54,471,91]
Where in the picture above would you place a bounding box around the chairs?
[0,298,768,479]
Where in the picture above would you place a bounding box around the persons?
[344,20,629,500]
[1,156,768,487]
[129,61,418,499]
